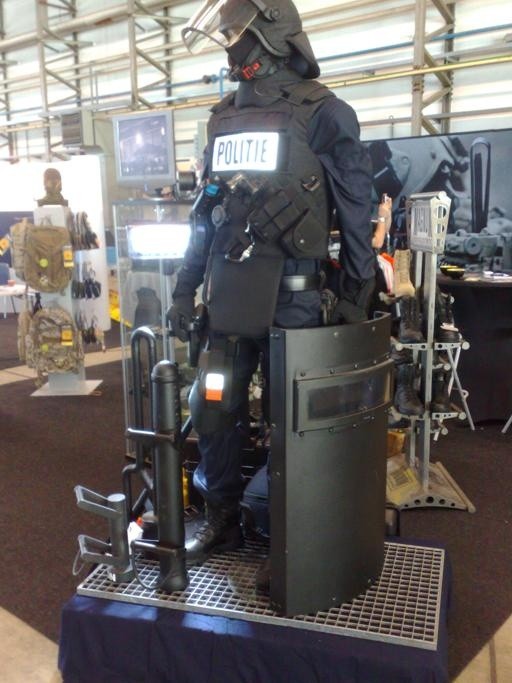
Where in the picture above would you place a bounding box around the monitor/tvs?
[123,218,191,264]
[113,109,178,188]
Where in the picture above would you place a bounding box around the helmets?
[216,0,319,79]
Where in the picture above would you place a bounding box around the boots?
[399,295,426,344]
[393,363,425,415]
[433,292,459,343]
[392,248,416,297]
[430,367,452,412]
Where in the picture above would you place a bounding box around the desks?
[55,536,453,679]
[0,283,36,319]
[438,273,511,425]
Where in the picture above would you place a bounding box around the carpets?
[0,311,133,371]
[0,347,512,683]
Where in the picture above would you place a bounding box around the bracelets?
[376,216,386,223]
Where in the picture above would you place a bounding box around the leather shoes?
[185,515,245,566]
[256,556,269,596]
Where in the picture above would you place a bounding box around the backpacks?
[16,291,84,385]
[23,216,74,293]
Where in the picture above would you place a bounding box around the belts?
[278,274,320,292]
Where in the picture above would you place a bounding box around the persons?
[372,190,392,319]
[163,0,373,585]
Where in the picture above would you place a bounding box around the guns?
[188,304,207,367]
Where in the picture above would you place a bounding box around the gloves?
[166,296,194,341]
